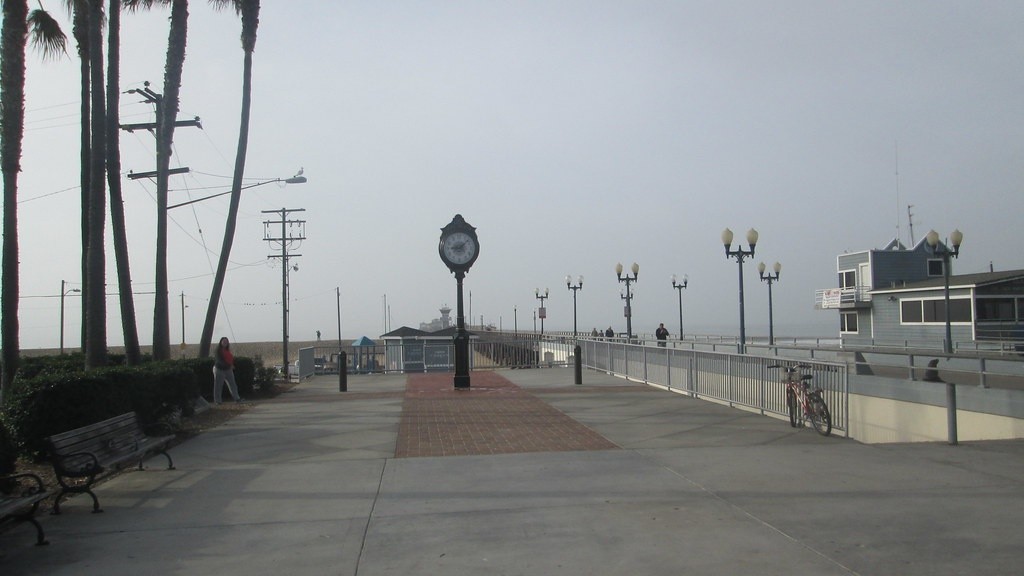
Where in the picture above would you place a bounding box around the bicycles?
[767,364,832,436]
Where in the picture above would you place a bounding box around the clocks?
[438,214,483,273]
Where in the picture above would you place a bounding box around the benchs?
[0,466,56,550]
[40,407,179,515]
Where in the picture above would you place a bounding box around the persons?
[655,323,671,348]
[317,331,321,340]
[591,328,604,341]
[605,326,614,341]
[212,337,244,405]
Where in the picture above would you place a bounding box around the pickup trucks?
[616,332,639,346]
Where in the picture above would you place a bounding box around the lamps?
[888,296,897,302]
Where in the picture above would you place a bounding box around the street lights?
[616,261,640,337]
[758,260,781,344]
[671,273,688,340]
[565,274,584,336]
[925,227,964,351]
[285,252,299,381]
[535,286,549,333]
[153,167,308,361]
[60,280,81,353]
[180,291,190,346]
[722,225,759,354]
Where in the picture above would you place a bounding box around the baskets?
[780,367,801,384]
